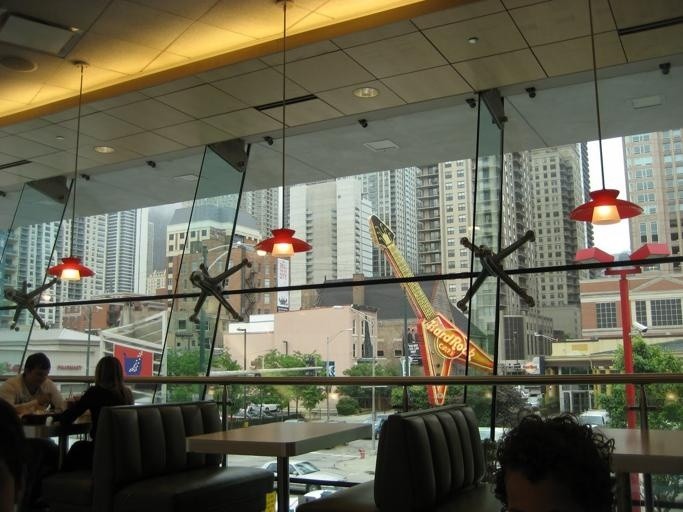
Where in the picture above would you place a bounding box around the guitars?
[367,214,495,405]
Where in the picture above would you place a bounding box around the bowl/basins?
[23,414,48,425]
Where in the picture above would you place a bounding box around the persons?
[0,352,65,512]
[491,414,616,512]
[58,356,133,471]
[0,397,29,512]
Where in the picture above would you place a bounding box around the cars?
[361,415,388,439]
[255,460,347,495]
[256,403,282,413]
[576,409,608,428]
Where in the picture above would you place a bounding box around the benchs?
[39,397,275,512]
[300,404,506,510]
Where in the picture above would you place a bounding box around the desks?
[596,428,682,512]
[183,411,376,511]
[22,418,93,472]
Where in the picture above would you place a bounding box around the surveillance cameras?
[632,322,648,332]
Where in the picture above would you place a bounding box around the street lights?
[197,241,268,401]
[324,328,353,422]
[235,324,247,421]
[333,303,378,450]
[573,243,672,512]
[42,292,94,393]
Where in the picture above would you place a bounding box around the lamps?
[254,1,314,258]
[569,1,643,226]
[45,56,98,284]
